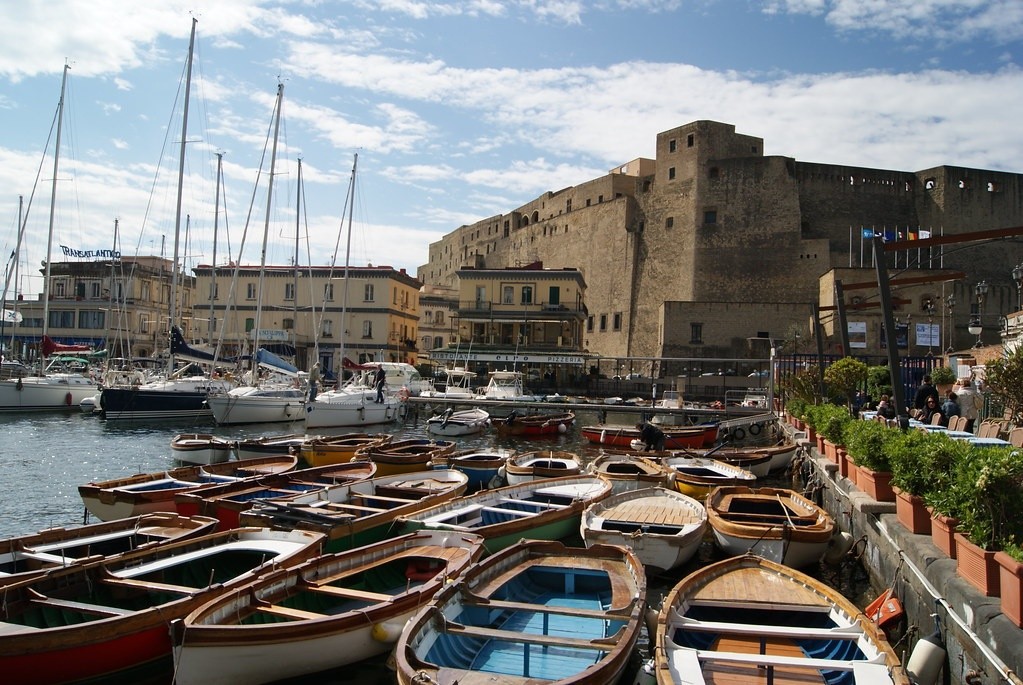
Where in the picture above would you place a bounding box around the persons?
[877,394,895,419]
[942,389,960,419]
[373,364,386,404]
[915,395,949,427]
[955,379,983,433]
[856,390,865,406]
[915,375,939,408]
[309,362,321,401]
[636,422,665,451]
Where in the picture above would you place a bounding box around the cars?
[612,368,771,380]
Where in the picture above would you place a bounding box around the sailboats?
[0,10,435,429]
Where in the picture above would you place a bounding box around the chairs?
[987,424,1001,438]
[860,411,865,420]
[866,410,872,412]
[948,414,959,430]
[1007,428,1023,446]
[878,415,888,424]
[956,416,968,431]
[913,424,928,435]
[978,422,990,437]
[930,412,941,425]
[982,407,1013,423]
[910,408,918,417]
[888,419,897,427]
[872,414,878,420]
[992,417,1018,439]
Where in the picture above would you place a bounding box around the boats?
[420,334,767,425]
[395,537,648,685]
[652,548,909,685]
[0,511,328,685]
[170,529,486,685]
[491,409,575,438]
[581,420,721,449]
[427,405,490,435]
[78,432,839,575]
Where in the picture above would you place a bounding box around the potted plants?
[766,378,1023,629]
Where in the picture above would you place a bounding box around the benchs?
[104,574,201,594]
[674,612,858,669]
[255,601,330,619]
[201,470,241,480]
[267,487,304,494]
[673,642,880,685]
[166,475,199,486]
[448,621,616,651]
[303,581,392,601]
[666,649,705,685]
[30,596,137,615]
[465,592,629,620]
[504,497,567,507]
[721,510,815,521]
[18,549,75,565]
[321,496,383,512]
[853,662,894,685]
[477,503,538,517]
[350,491,411,503]
[292,478,332,487]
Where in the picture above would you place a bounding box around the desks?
[858,412,879,415]
[925,428,975,438]
[909,423,947,428]
[909,418,921,424]
[865,414,877,421]
[950,436,1012,445]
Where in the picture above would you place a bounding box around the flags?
[863,229,930,243]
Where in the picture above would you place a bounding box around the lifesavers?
[723,434,734,447]
[734,428,746,440]
[405,560,446,580]
[749,424,761,435]
[400,387,409,401]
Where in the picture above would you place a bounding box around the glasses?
[926,400,935,404]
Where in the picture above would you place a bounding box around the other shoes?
[309,399,317,402]
[379,401,384,404]
[373,399,378,403]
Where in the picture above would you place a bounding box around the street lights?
[1012,263,1023,312]
[905,314,913,357]
[794,331,801,356]
[945,294,956,352]
[972,280,988,348]
[926,301,935,358]
[968,313,1008,360]
